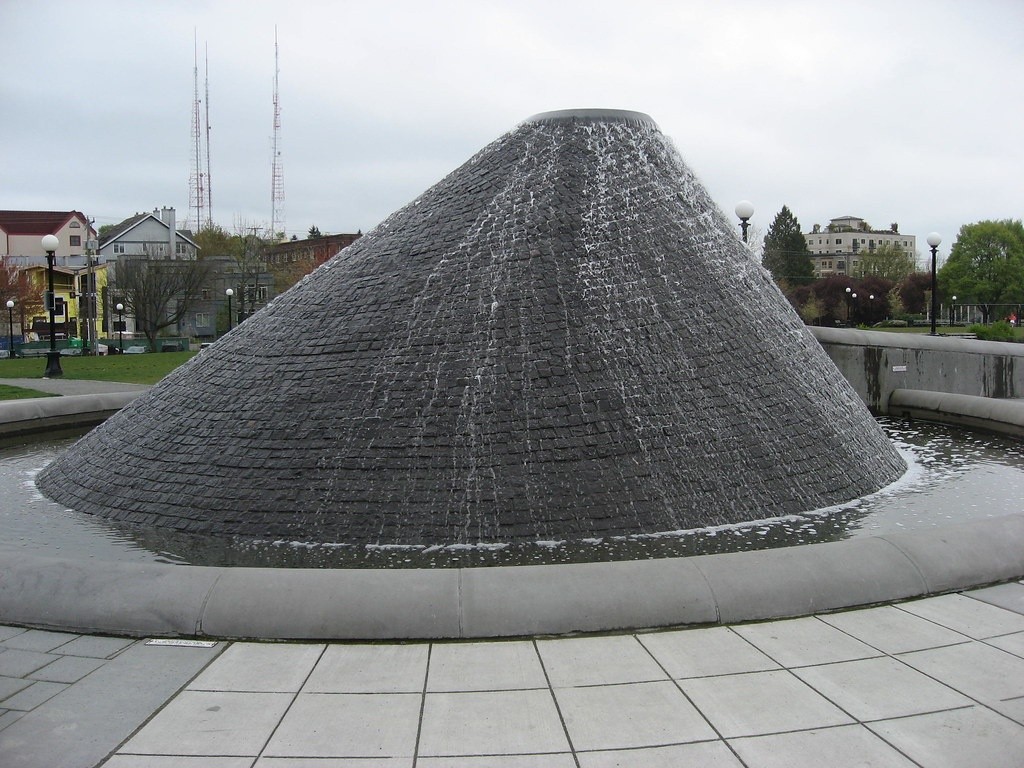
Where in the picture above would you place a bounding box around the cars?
[162,343,184,351]
[0,349,20,359]
[60,349,77,356]
[200,343,213,351]
[125,345,147,353]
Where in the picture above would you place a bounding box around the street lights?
[7,301,15,359]
[852,293,857,327]
[42,234,63,376]
[225,289,233,330]
[952,296,957,326]
[735,200,755,243]
[846,287,851,326]
[870,295,874,328]
[116,303,124,354]
[926,231,941,334]
